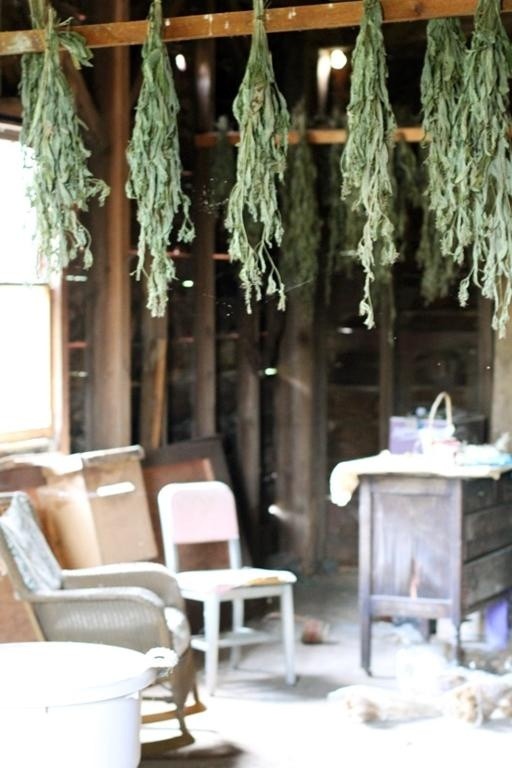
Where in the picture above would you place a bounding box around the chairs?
[157,479,297,698]
[0,491,206,755]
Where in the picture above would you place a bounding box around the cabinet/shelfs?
[329,448,512,676]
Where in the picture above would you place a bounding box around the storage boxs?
[40,444,160,571]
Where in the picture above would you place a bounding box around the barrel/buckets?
[419,391,455,453]
[388,415,415,455]
